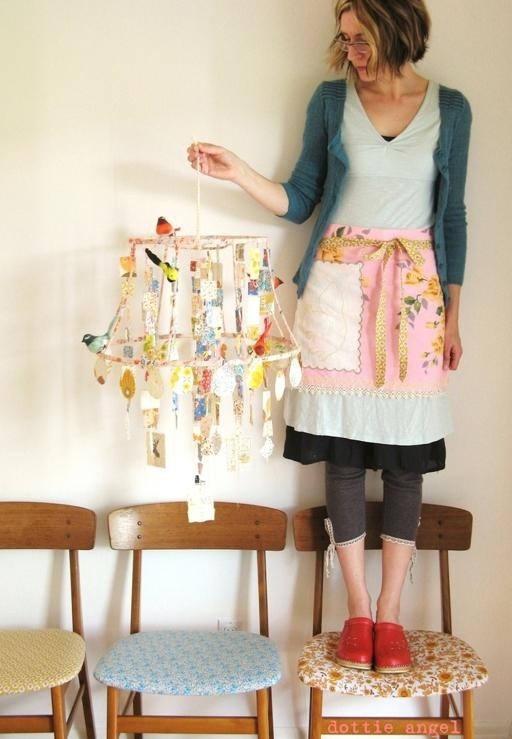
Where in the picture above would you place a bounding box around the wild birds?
[254,318,272,357]
[250,275,284,291]
[194,396,206,422]
[157,216,181,237]
[144,247,178,283]
[81,314,116,356]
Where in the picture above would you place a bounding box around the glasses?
[334,39,371,53]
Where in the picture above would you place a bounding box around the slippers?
[336,618,374,670]
[373,622,411,674]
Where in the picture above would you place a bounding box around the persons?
[188,3,473,678]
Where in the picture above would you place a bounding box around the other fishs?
[119,256,137,278]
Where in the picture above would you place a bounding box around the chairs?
[0,499,99,739]
[289,501,490,739]
[92,499,289,738]
